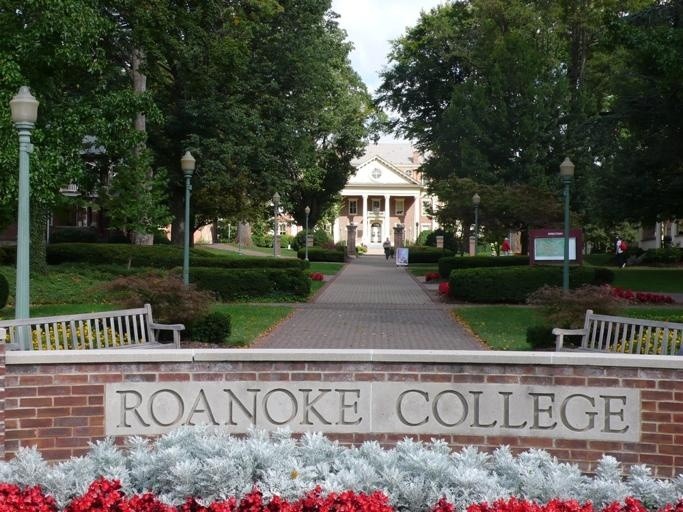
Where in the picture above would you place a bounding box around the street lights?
[272,191,281,258]
[559,157,575,289]
[9,85,40,349]
[181,151,197,285]
[471,193,481,256]
[304,206,310,262]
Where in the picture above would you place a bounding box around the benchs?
[552,309,683,355]
[0,303,185,351]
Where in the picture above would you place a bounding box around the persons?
[381,237,391,262]
[614,236,629,269]
[500,236,510,256]
[389,246,395,260]
